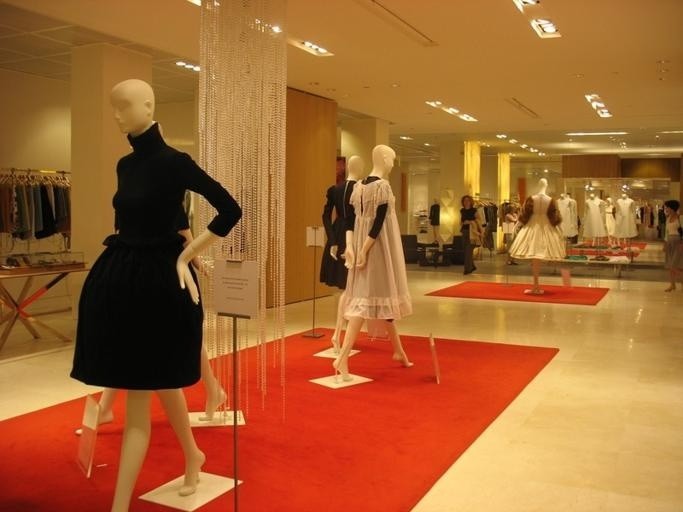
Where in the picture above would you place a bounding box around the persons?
[509,177,563,297]
[332,145,425,382]
[319,144,381,355]
[90,114,228,432]
[605,194,617,234]
[429,197,444,246]
[564,193,581,234]
[657,197,683,292]
[458,197,487,273]
[582,191,610,257]
[72,79,245,512]
[553,189,572,242]
[613,190,643,256]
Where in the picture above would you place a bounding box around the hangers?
[0,169,71,186]
[470,196,498,209]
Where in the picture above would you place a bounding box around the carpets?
[578,243,646,249]
[0,326,561,512]
[423,280,610,307]
[565,249,639,258]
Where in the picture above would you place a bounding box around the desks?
[0,263,89,347]
